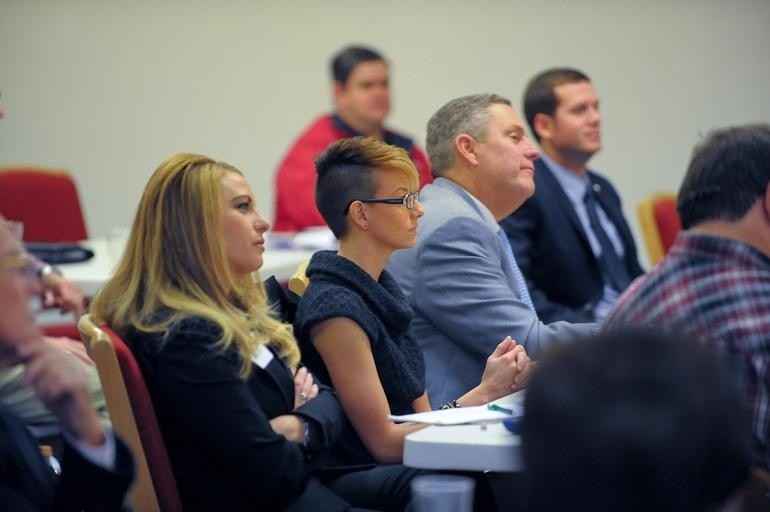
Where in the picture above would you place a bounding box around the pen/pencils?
[488,405,513,414]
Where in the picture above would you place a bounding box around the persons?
[82,153,498,510]
[288,134,537,512]
[515,321,755,511]
[0,222,112,435]
[495,66,649,325]
[379,92,606,412]
[600,120,770,512]
[268,46,433,250]
[0,215,140,509]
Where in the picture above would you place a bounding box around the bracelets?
[35,261,53,283]
[438,398,461,409]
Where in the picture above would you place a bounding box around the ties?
[584,194,632,293]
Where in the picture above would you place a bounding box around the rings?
[297,391,307,401]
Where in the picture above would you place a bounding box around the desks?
[403,384,530,471]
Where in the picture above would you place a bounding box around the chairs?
[639,194,681,266]
[288,258,310,296]
[78,308,184,512]
[1,164,112,335]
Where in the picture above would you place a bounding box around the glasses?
[342,191,419,216]
